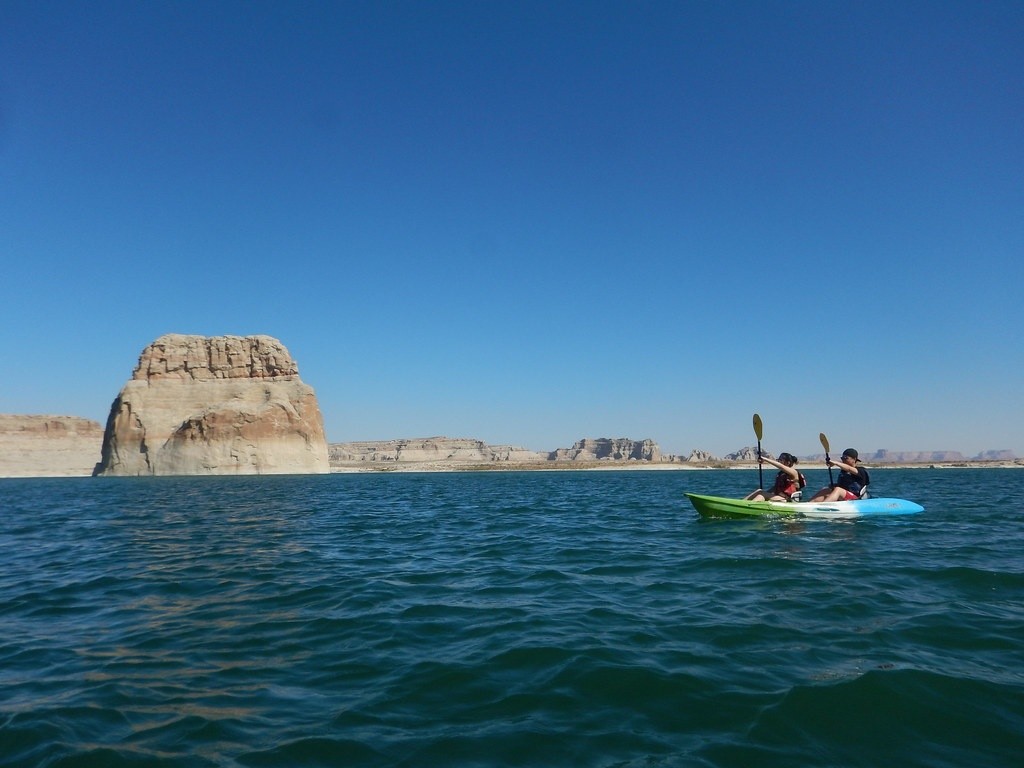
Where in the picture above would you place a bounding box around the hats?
[843,448,861,462]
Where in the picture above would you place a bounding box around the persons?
[743,453,799,503]
[807,448,866,502]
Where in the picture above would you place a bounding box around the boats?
[684,492,925,518]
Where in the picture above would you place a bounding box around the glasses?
[775,459,785,462]
[841,457,851,460]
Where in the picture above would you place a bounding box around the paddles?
[751,413,764,492]
[819,432,834,489]
